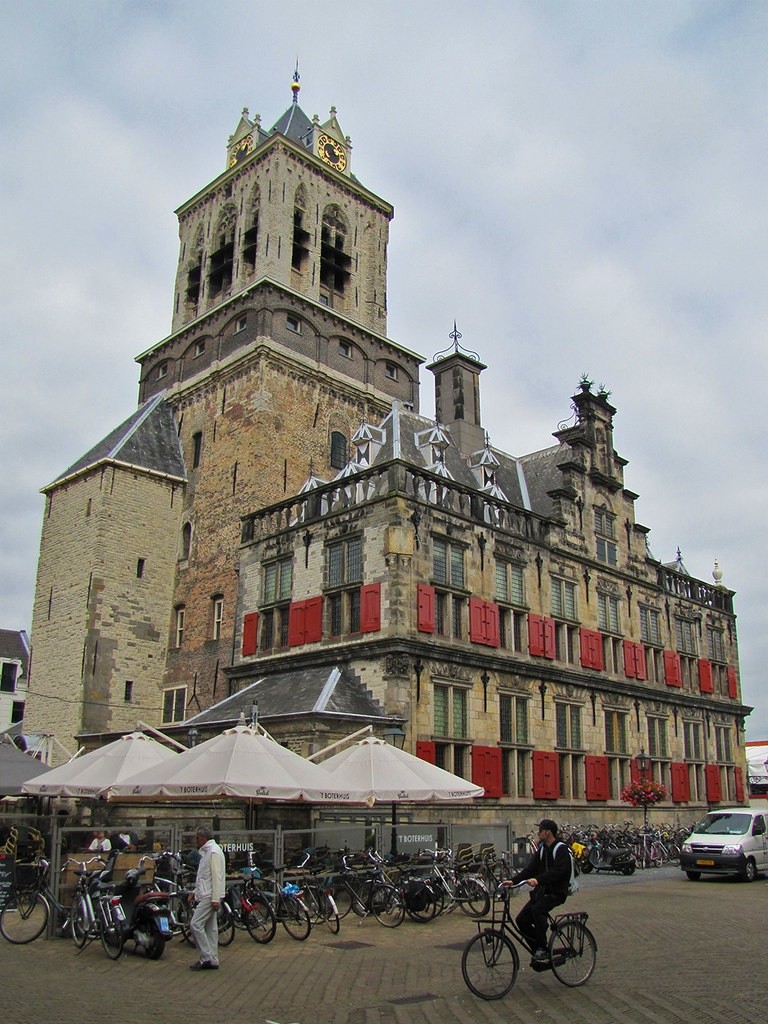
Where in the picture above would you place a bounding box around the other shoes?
[533,948,550,963]
[190,960,218,970]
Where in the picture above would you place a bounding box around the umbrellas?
[0,724,485,862]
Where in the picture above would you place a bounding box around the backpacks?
[540,842,582,896]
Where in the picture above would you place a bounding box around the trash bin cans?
[513,836,538,869]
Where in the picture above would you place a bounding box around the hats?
[534,819,557,833]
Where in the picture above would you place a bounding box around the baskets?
[15,864,48,886]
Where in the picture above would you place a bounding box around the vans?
[678,808,768,882]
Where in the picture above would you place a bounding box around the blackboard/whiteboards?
[0,854,17,912]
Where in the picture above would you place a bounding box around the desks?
[15,861,44,892]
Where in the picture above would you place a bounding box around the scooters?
[582,836,637,876]
[115,860,176,961]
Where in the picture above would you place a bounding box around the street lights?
[635,746,650,865]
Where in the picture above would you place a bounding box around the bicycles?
[462,878,597,1001]
[0,818,703,962]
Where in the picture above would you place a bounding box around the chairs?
[452,839,495,891]
[5,824,45,862]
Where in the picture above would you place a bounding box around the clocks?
[316,133,347,171]
[229,133,254,168]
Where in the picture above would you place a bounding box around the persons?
[116,826,131,851]
[105,831,113,849]
[188,827,225,970]
[88,831,112,853]
[502,819,572,968]
[126,823,138,850]
